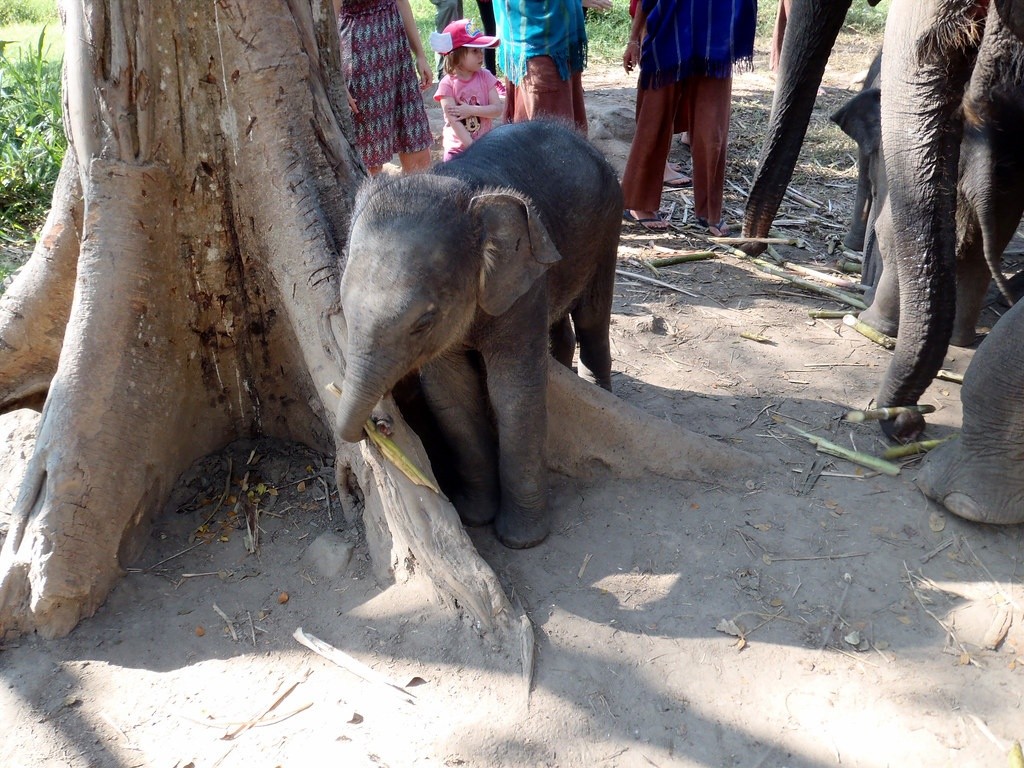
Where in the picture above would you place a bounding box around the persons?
[429,0,496,82]
[621,0,758,239]
[493,0,613,140]
[333,0,434,179]
[433,19,502,161]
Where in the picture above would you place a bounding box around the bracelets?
[628,41,638,45]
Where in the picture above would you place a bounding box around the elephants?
[339,119,625,549]
[831,53,1023,347]
[881,2,1023,525]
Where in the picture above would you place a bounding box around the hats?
[429,19,500,54]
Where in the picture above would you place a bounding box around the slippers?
[623,209,669,233]
[696,216,732,236]
